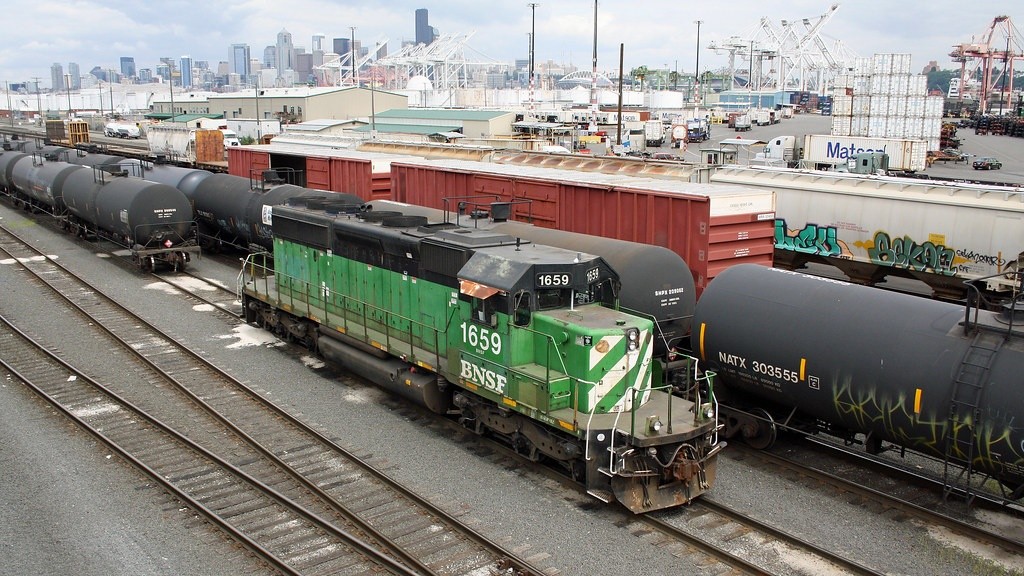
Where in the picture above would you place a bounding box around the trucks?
[728,112,751,132]
[645,120,666,147]
[688,118,711,142]
[757,110,782,126]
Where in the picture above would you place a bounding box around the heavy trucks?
[756,134,929,176]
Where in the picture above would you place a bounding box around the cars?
[973,158,1002,170]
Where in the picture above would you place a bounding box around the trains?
[238,190,727,515]
